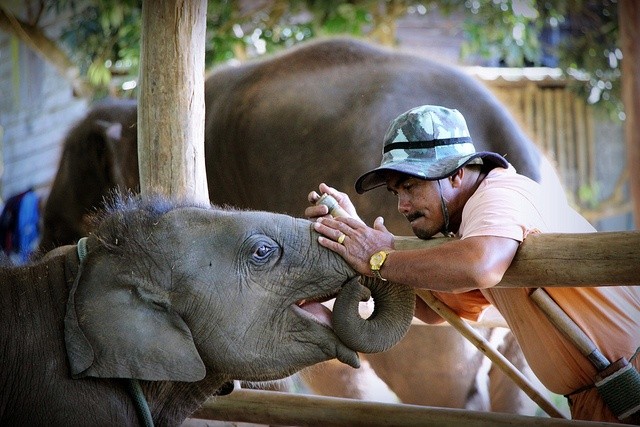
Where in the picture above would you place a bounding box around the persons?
[305,104,639,426]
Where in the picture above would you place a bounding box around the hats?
[355,104,509,194]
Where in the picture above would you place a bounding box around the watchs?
[369,247,395,282]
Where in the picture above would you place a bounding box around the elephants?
[0,184,418,422]
[36,33,571,417]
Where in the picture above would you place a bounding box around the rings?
[337,234,345,243]
